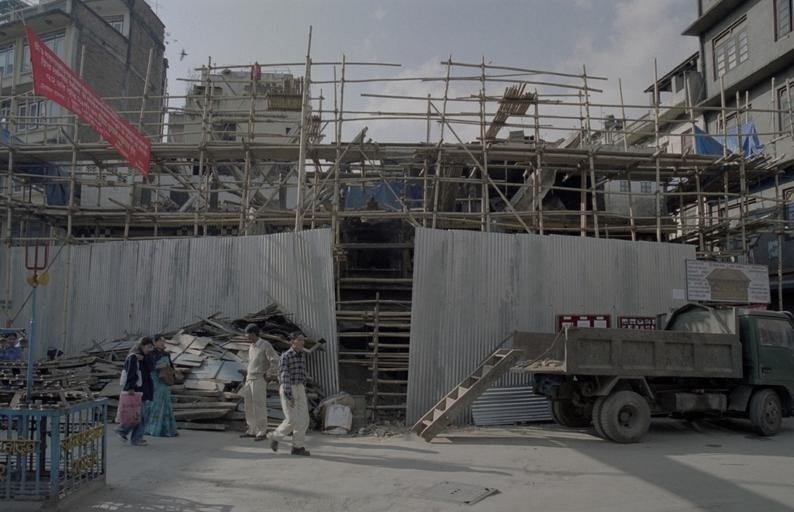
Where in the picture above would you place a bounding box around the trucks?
[509,300,794,444]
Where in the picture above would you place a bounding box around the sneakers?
[114,430,148,446]
[271,440,278,452]
[240,433,266,441]
[291,447,311,456]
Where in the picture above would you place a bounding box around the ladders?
[412,347,522,443]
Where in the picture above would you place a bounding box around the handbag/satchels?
[115,390,142,426]
[119,352,142,387]
[158,366,174,386]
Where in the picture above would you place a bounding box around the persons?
[111,333,180,446]
[266,333,310,456]
[238,323,280,442]
